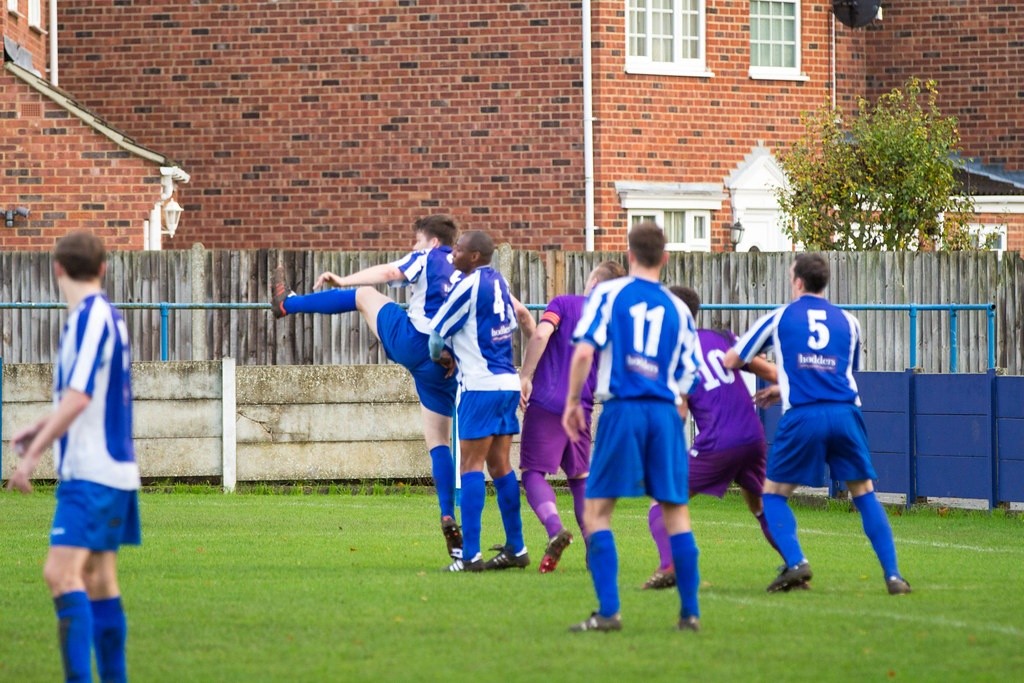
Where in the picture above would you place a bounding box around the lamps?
[161,197,184,238]
[723,218,745,252]
[0,206,30,228]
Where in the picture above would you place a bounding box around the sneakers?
[643,562,677,590]
[269,265,297,319]
[886,575,911,595]
[766,563,813,594]
[539,529,573,574]
[567,610,623,633]
[441,515,463,560]
[483,544,531,570]
[673,617,700,632]
[442,551,484,573]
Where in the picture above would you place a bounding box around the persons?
[427,228,536,576]
[642,285,810,588]
[519,260,628,573]
[4,230,141,683]
[273,214,464,564]
[561,220,702,631]
[723,255,912,595]
